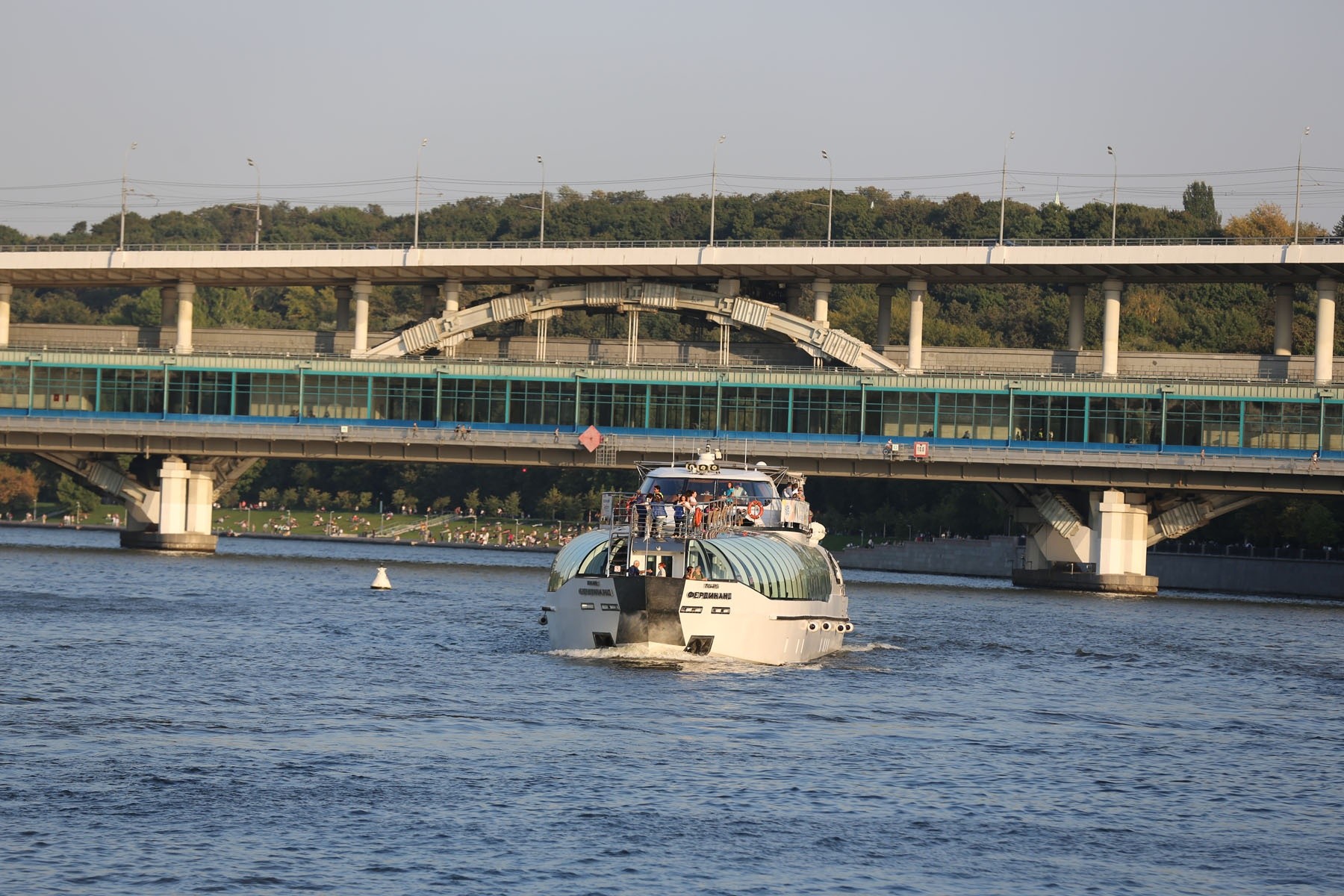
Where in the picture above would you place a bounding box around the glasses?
[797,490,802,491]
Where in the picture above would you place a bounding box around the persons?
[885,438,894,453]
[454,423,473,441]
[412,423,418,438]
[629,481,750,540]
[289,409,330,418]
[781,482,813,530]
[623,560,708,582]
[553,428,559,444]
[0,472,592,551]
[923,428,934,437]
[1200,449,1205,466]
[1016,427,1054,441]
[962,430,970,439]
[1312,452,1320,469]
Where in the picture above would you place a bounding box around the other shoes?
[681,535,685,538]
[671,536,675,537]
[674,535,677,538]
[655,534,661,538]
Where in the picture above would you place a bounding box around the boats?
[537,438,854,668]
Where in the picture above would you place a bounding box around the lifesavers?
[748,500,763,519]
[695,507,702,526]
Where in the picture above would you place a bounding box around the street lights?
[424,515,428,542]
[1294,125,1310,245]
[245,507,250,531]
[120,141,138,249]
[1107,145,1117,246]
[473,517,477,534]
[537,154,546,248]
[76,502,80,524]
[34,499,37,522]
[329,511,335,536]
[287,510,290,527]
[710,133,727,247]
[557,519,561,544]
[247,156,261,251]
[414,139,428,248]
[999,129,1015,247]
[821,148,834,248]
[380,513,385,538]
[515,519,519,544]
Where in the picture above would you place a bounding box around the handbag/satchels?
[784,490,790,498]
